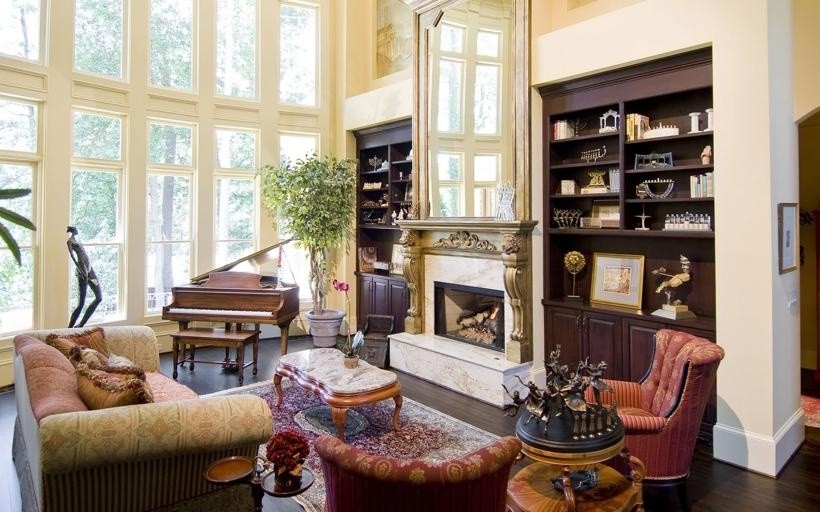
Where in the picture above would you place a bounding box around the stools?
[167,327,262,386]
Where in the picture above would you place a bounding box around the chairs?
[580,327,726,491]
[311,422,522,512]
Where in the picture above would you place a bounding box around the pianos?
[162,239,299,379]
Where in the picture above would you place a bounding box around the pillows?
[48,324,110,358]
[78,345,110,369]
[106,350,133,369]
[74,366,153,410]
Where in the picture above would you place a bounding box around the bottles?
[391,210,398,226]
[663,211,711,231]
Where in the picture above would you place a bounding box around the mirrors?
[403,1,532,219]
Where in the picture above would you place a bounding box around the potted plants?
[257,150,366,349]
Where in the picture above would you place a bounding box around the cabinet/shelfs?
[388,274,410,333]
[353,272,388,332]
[353,119,412,230]
[622,311,716,445]
[537,40,714,239]
[545,293,622,381]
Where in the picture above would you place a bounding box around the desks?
[507,457,636,511]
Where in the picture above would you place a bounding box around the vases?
[343,355,359,369]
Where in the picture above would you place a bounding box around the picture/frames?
[403,182,412,203]
[589,251,647,311]
[389,243,406,276]
[778,202,800,275]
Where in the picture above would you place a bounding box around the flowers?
[332,278,363,358]
[263,428,311,487]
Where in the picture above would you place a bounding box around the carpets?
[200,374,503,512]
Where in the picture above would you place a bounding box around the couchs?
[11,323,275,512]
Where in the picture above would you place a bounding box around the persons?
[63,225,103,327]
[500,345,616,438]
